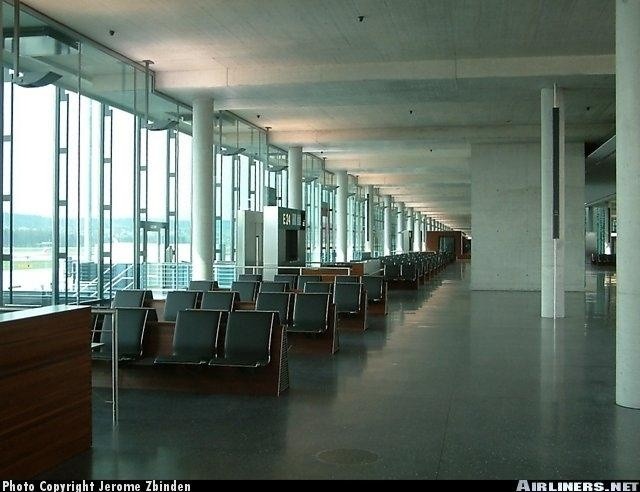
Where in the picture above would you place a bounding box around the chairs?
[207,310,275,368]
[159,291,198,322]
[380,251,450,291]
[91,309,150,364]
[303,282,332,293]
[111,289,147,310]
[333,276,362,304]
[238,274,261,282]
[273,274,297,290]
[259,281,286,293]
[188,281,215,291]
[590,252,616,266]
[333,283,362,314]
[254,292,291,329]
[154,310,222,366]
[200,291,236,311]
[299,276,320,291]
[277,267,301,275]
[362,275,383,303]
[285,293,330,335]
[230,282,254,301]
